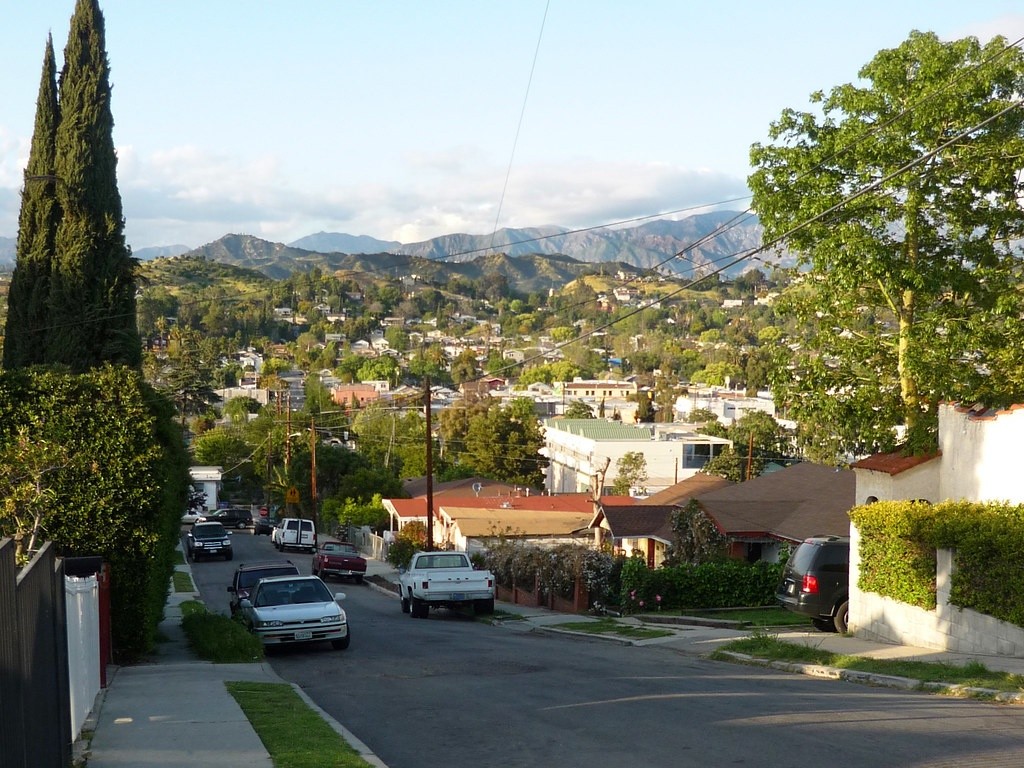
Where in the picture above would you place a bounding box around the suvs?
[774,533,850,634]
[195,508,253,529]
[228,560,302,621]
[188,522,233,562]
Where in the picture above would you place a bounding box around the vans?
[275,518,317,552]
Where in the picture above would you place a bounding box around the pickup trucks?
[254,517,275,536]
[397,553,495,618]
[311,542,366,583]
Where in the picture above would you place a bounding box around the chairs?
[264,588,284,605]
[297,587,316,600]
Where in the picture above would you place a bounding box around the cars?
[181,510,202,524]
[240,575,351,653]
